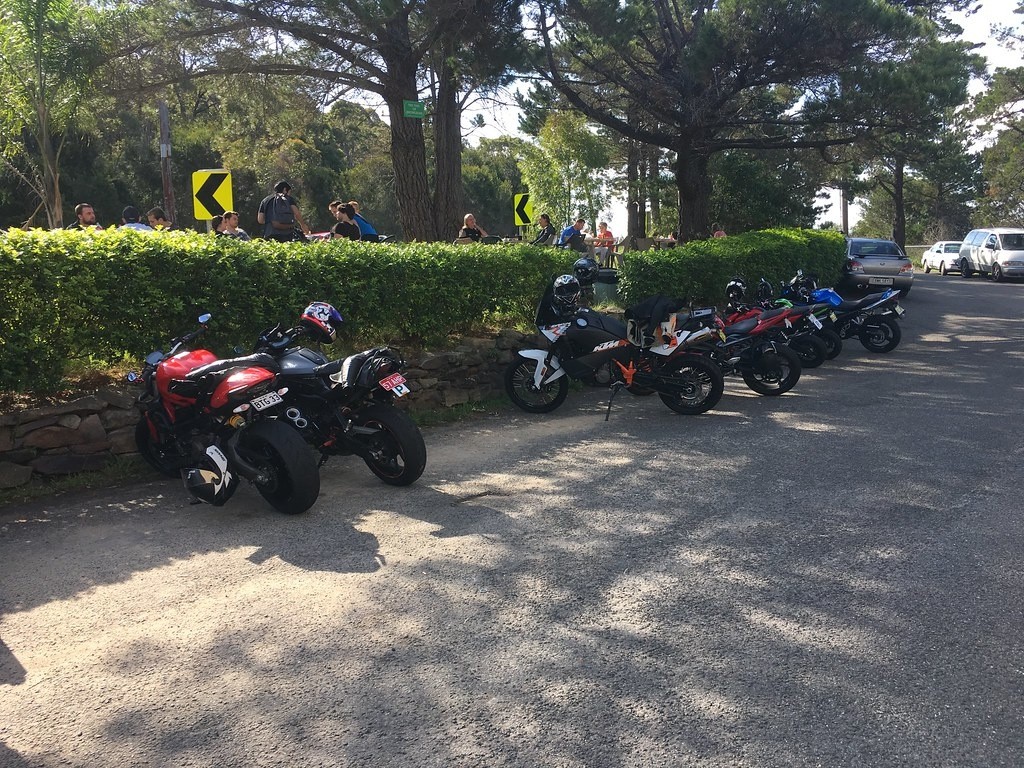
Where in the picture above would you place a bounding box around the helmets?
[572,258,599,286]
[553,275,582,308]
[725,278,747,301]
[180,445,240,507]
[299,302,344,345]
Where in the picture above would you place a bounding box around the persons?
[711,222,726,237]
[529,214,555,246]
[458,213,488,244]
[117,206,152,230]
[593,222,615,269]
[65,203,103,231]
[212,216,227,238]
[258,181,308,243]
[223,211,252,241]
[328,200,379,243]
[145,207,181,231]
[667,231,678,249]
[558,219,585,251]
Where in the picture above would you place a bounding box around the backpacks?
[271,192,294,230]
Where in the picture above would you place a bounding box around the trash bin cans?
[591,267,619,304]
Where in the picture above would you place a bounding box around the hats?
[274,179,292,191]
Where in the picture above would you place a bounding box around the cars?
[842,238,914,296]
[921,240,988,276]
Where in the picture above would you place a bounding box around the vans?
[958,227,1024,282]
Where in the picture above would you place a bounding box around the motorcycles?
[679,292,816,396]
[505,258,725,421]
[714,279,842,369]
[128,312,321,515]
[234,302,427,487]
[780,270,907,353]
